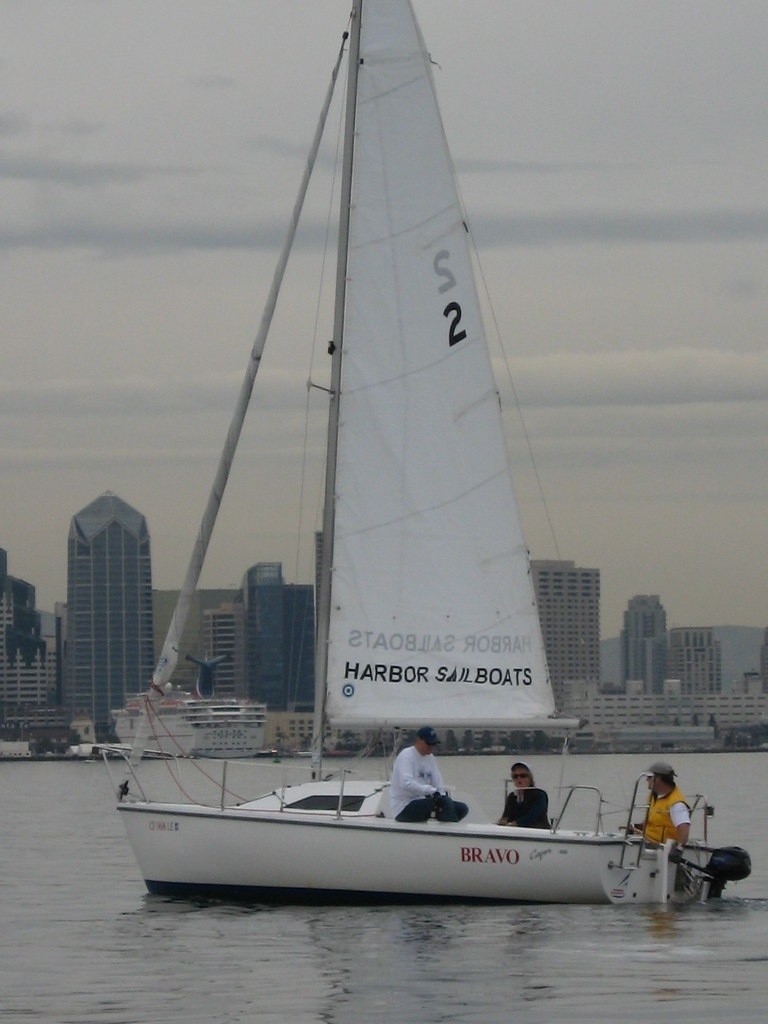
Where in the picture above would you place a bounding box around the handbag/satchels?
[436,795,458,822]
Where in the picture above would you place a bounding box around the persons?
[494,763,551,830]
[626,760,693,866]
[388,725,469,822]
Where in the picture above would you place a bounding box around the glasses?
[512,774,529,779]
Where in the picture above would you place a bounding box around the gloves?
[669,843,684,865]
[628,823,641,834]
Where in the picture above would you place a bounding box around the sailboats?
[92,0,751,907]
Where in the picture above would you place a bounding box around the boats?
[109,651,266,757]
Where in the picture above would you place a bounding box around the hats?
[511,762,530,771]
[649,762,677,777]
[417,727,440,745]
